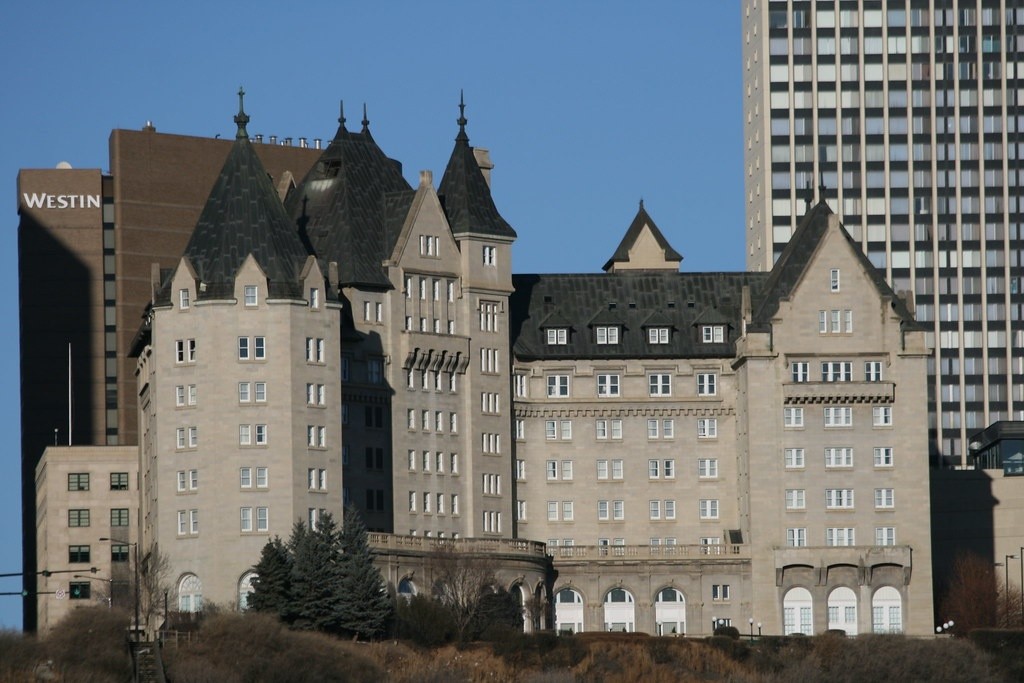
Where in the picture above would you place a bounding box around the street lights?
[991,563,1004,627]
[99,538,139,683]
[164,587,169,639]
[74,575,113,608]
[1006,555,1020,629]
